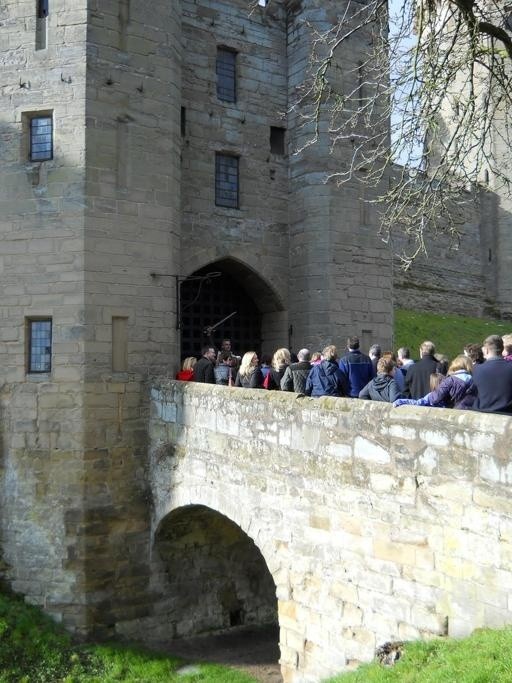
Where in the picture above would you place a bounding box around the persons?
[177,334,511,417]
